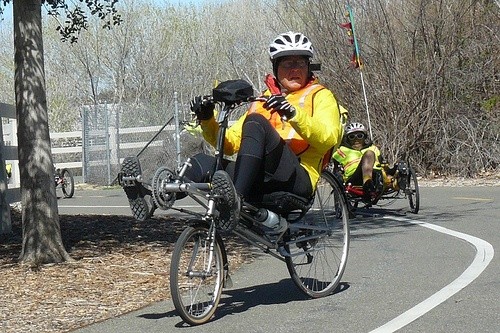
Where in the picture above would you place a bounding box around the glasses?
[348,133,364,140]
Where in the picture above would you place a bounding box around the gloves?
[189,95,215,120]
[263,93,296,122]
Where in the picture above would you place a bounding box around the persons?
[332,123,408,203]
[118,31,340,231]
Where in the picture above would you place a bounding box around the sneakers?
[363,176,374,191]
[212,170,240,233]
[393,160,407,191]
[118,156,155,222]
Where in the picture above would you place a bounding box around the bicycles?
[55,163,74,197]
[151,78,350,326]
[332,164,420,219]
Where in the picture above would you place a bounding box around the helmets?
[345,122,367,135]
[268,31,313,63]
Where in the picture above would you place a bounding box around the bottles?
[255,208,287,232]
